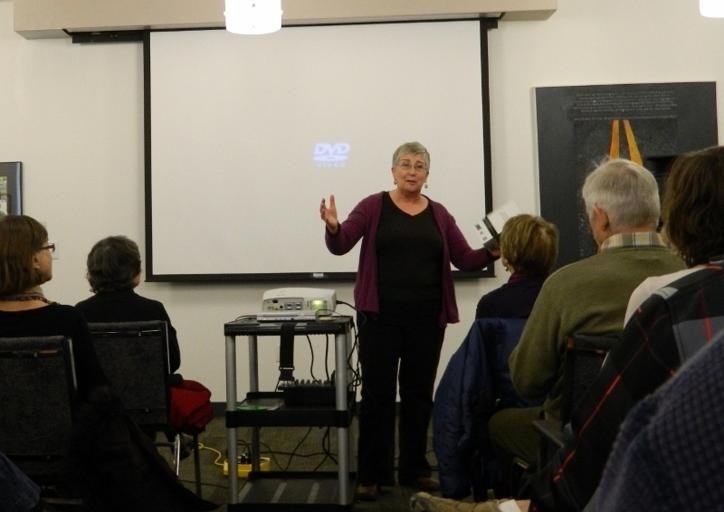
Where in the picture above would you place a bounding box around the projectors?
[255,286,337,316]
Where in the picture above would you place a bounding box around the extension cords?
[223,456,271,480]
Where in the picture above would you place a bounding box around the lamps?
[214,0,285,41]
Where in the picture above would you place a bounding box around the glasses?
[40,243,55,252]
[397,159,424,171]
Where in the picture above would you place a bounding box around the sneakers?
[163,470,227,512]
[411,477,439,491]
[409,492,509,512]
[355,484,378,501]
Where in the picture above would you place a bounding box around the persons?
[485,159,689,471]
[527,146,724,512]
[1,216,221,512]
[0,451,54,512]
[580,328,724,511]
[431,215,559,502]
[73,236,193,462]
[411,266,706,512]
[321,142,501,501]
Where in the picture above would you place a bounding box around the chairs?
[498,334,627,498]
[77,321,199,479]
[1,336,84,468]
